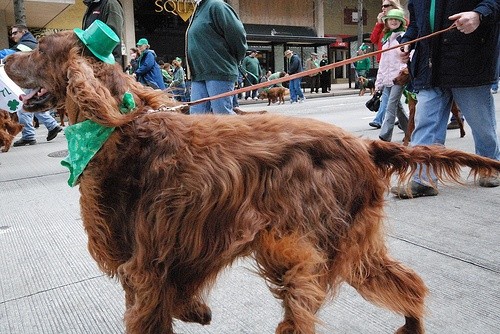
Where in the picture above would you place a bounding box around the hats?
[249,49,259,54]
[283,49,293,57]
[136,38,148,46]
[381,9,406,28]
[310,52,317,55]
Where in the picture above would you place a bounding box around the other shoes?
[394,121,399,125]
[478,176,500,188]
[368,122,380,129]
[391,179,438,198]
[47,125,62,141]
[292,96,306,103]
[13,138,36,146]
[246,97,258,100]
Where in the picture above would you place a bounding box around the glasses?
[10,31,20,36]
[380,4,392,8]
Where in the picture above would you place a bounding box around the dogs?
[57,106,69,127]
[257,87,285,106]
[390,66,465,147]
[0,29,500,334]
[357,76,375,96]
[0,108,23,153]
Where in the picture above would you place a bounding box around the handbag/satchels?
[365,90,382,112]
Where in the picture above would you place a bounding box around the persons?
[284,49,305,102]
[10,23,63,146]
[184,0,248,114]
[319,54,330,93]
[369,0,500,198]
[261,69,274,89]
[127,38,191,102]
[354,43,371,90]
[267,71,286,90]
[307,51,321,93]
[80,0,125,72]
[236,68,243,99]
[242,50,263,100]
